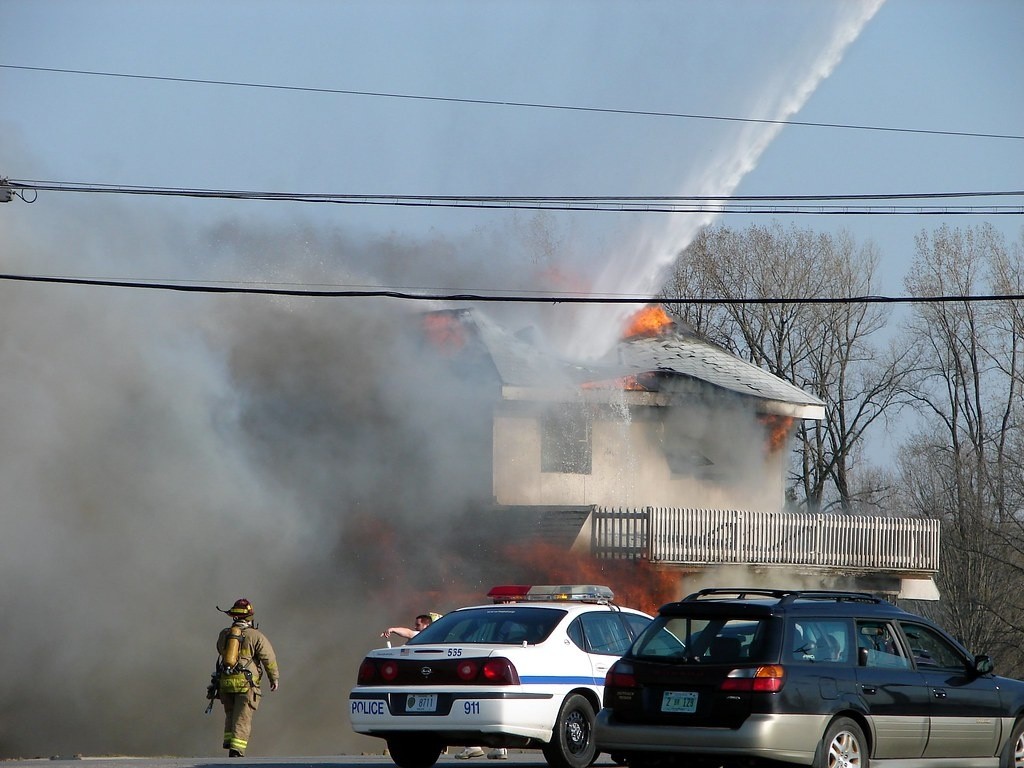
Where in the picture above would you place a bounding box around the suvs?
[590,586,1024,768]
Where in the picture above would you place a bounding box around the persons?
[215,600,279,758]
[379,614,510,761]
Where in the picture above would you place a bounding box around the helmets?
[226,597,255,618]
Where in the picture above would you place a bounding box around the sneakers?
[488,747,508,759]
[455,745,484,759]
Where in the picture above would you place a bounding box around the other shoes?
[226,749,246,759]
[222,739,230,749]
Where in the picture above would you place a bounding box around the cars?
[348,585,698,768]
